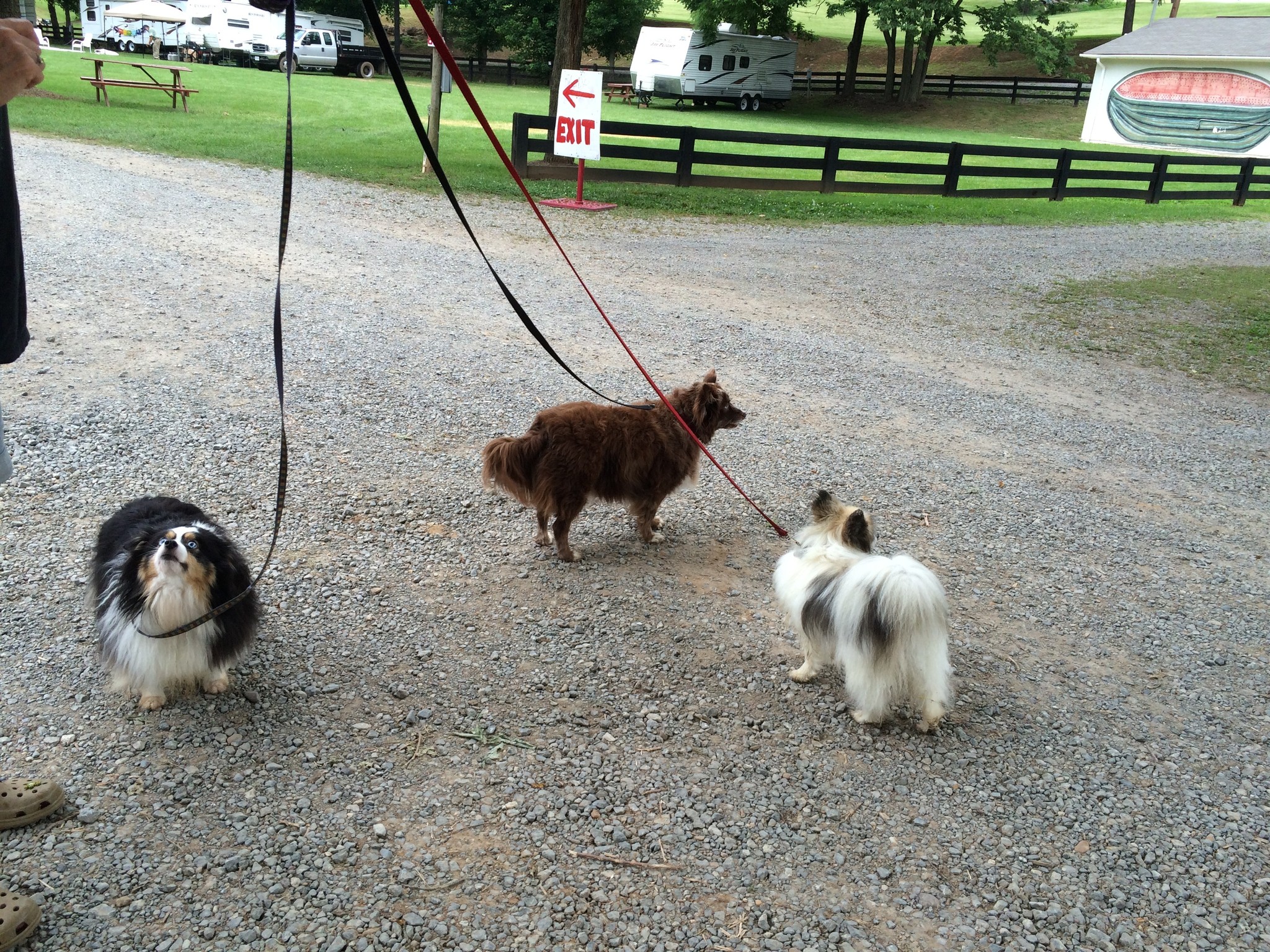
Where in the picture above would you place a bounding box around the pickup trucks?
[249,27,385,79]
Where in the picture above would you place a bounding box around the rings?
[34,53,44,66]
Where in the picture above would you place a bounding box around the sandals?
[0,890,44,951]
[0,777,66,830]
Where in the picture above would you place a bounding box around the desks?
[81,56,193,112]
[221,47,251,68]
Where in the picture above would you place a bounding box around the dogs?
[481,368,749,563]
[770,490,960,732]
[87,495,267,711]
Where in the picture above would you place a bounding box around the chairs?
[34,28,50,48]
[71,32,93,54]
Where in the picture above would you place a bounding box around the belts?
[153,38,162,40]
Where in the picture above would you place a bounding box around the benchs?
[79,75,186,88]
[89,80,199,95]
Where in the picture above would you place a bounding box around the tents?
[104,0,186,61]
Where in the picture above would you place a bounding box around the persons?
[142,25,162,60]
[0,0,46,480]
